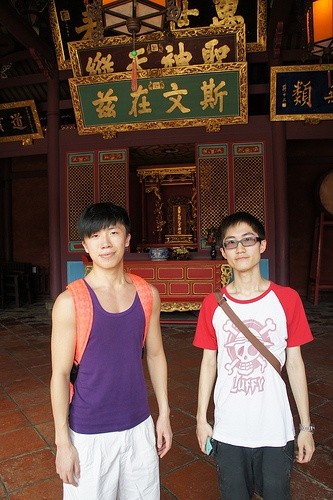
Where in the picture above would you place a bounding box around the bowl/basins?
[149,248,169,261]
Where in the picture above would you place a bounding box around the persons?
[192,212,315,500]
[50,201,173,500]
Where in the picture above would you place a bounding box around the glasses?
[222,236,265,249]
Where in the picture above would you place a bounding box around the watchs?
[299,422,315,434]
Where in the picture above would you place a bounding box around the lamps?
[307,0,333,66]
